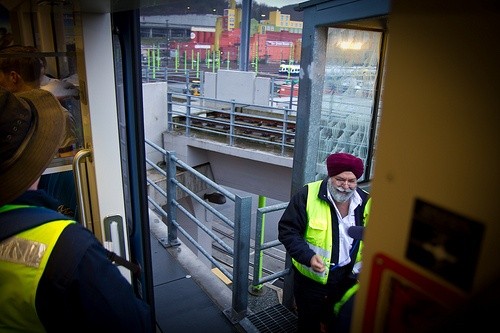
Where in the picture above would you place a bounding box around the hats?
[326,154,364,179]
[0,86,67,208]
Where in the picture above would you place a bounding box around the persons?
[0,28,150,333]
[278,154,371,333]
